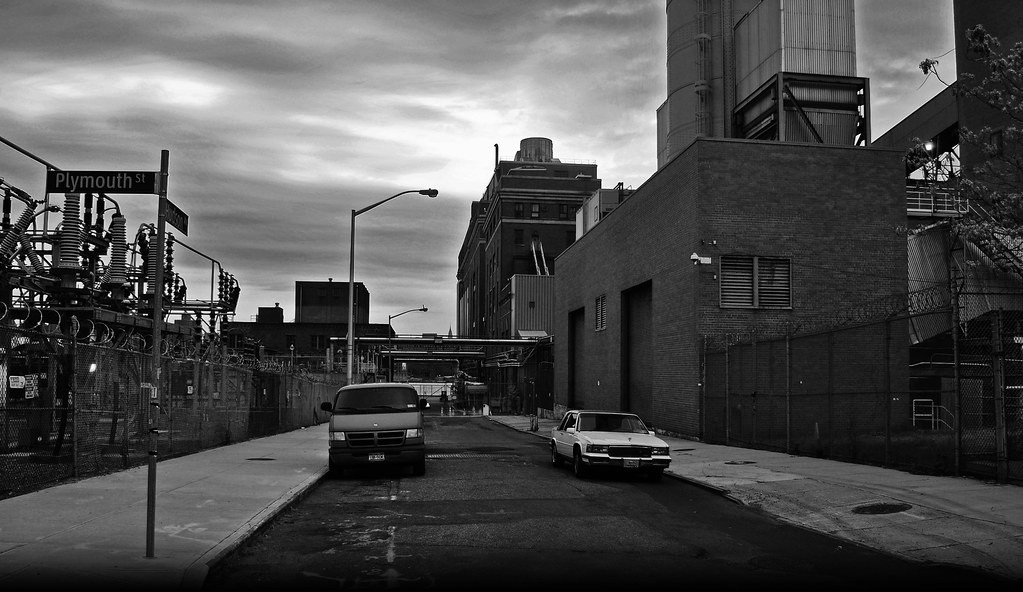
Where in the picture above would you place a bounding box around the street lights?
[388,304,428,382]
[346,188,438,386]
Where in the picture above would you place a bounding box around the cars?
[549,409,672,482]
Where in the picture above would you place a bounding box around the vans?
[321,382,429,475]
